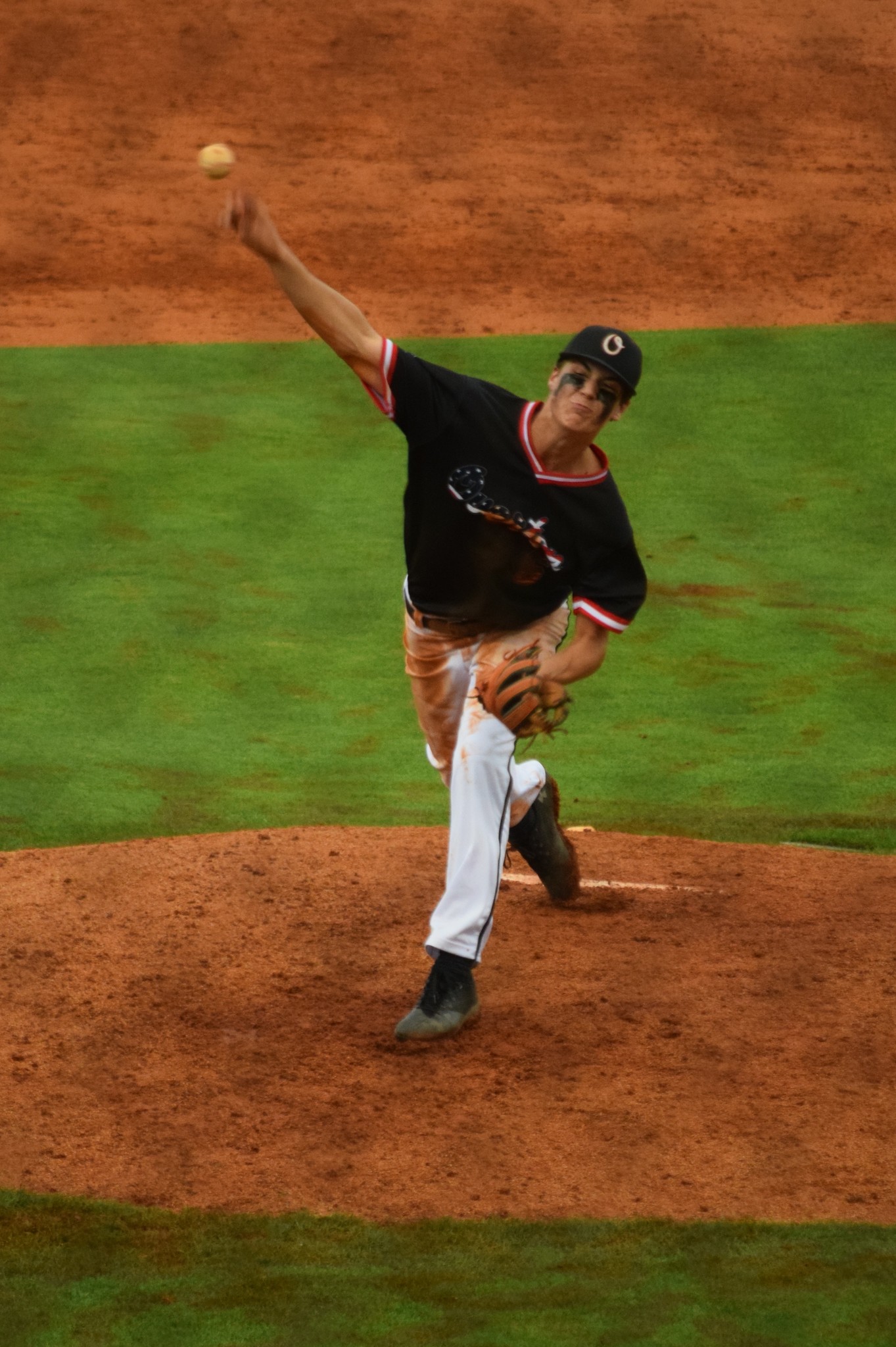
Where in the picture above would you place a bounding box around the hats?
[559,324,643,396]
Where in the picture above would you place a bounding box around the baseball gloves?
[466,636,576,755]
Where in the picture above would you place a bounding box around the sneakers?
[391,963,481,1040]
[508,771,577,901]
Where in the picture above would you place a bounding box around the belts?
[403,590,508,637]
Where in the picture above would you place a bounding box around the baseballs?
[198,143,236,179]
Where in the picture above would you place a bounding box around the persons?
[218,187,647,1038]
